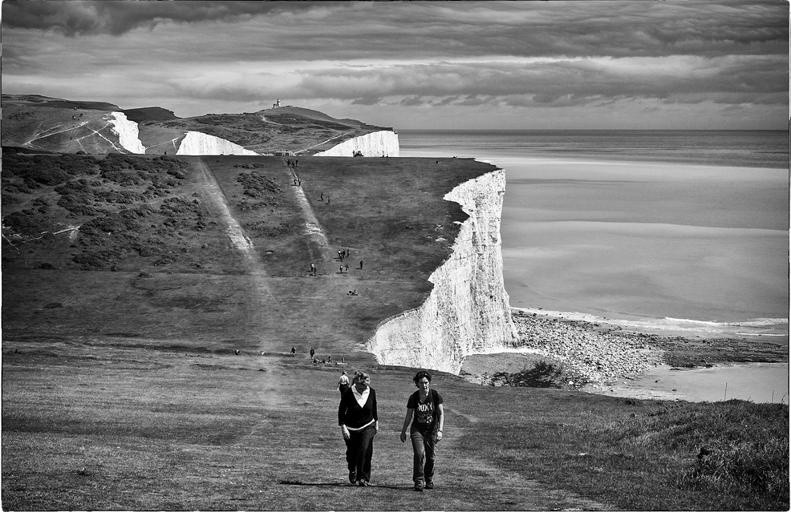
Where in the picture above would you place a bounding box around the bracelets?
[438,430,444,432]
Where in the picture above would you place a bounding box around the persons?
[401,371,444,491]
[287,158,302,186]
[310,347,333,367]
[311,263,316,277]
[310,247,364,296]
[319,191,330,206]
[292,345,296,357]
[338,370,378,487]
[338,372,350,400]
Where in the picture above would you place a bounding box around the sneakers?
[349,472,356,484]
[359,479,368,486]
[426,481,433,489]
[415,480,424,491]
